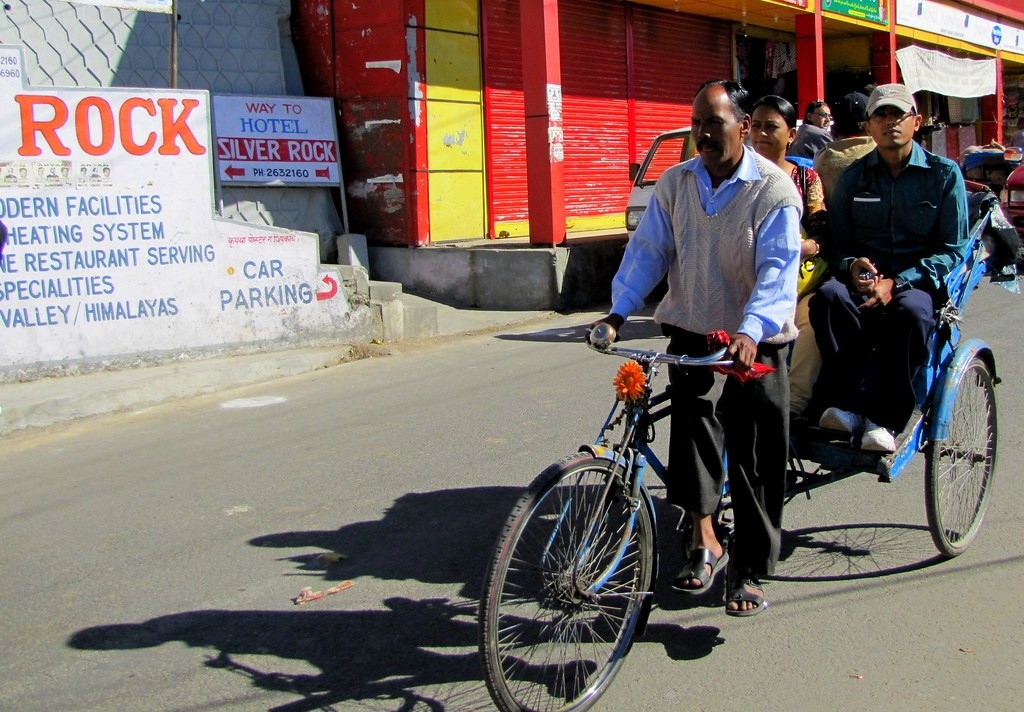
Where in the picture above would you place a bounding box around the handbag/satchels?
[797,223,826,302]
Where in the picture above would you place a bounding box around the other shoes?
[790,413,809,426]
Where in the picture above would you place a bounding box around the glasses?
[812,112,833,120]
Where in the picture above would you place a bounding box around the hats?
[844,91,869,121]
[865,83,917,115]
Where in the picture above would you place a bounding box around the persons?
[750,94,832,429]
[787,100,835,162]
[812,91,880,209]
[1010,116,1024,149]
[580,79,805,621]
[806,84,971,455]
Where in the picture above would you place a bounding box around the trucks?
[625,119,835,296]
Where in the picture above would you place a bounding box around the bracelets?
[807,238,821,259]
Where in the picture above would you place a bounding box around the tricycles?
[477,180,1003,712]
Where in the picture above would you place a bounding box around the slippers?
[671,548,729,595]
[726,579,766,616]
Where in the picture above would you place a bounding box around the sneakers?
[861,416,896,451]
[819,407,862,442]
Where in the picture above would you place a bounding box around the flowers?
[614,362,646,402]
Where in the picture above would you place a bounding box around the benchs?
[800,179,991,403]
